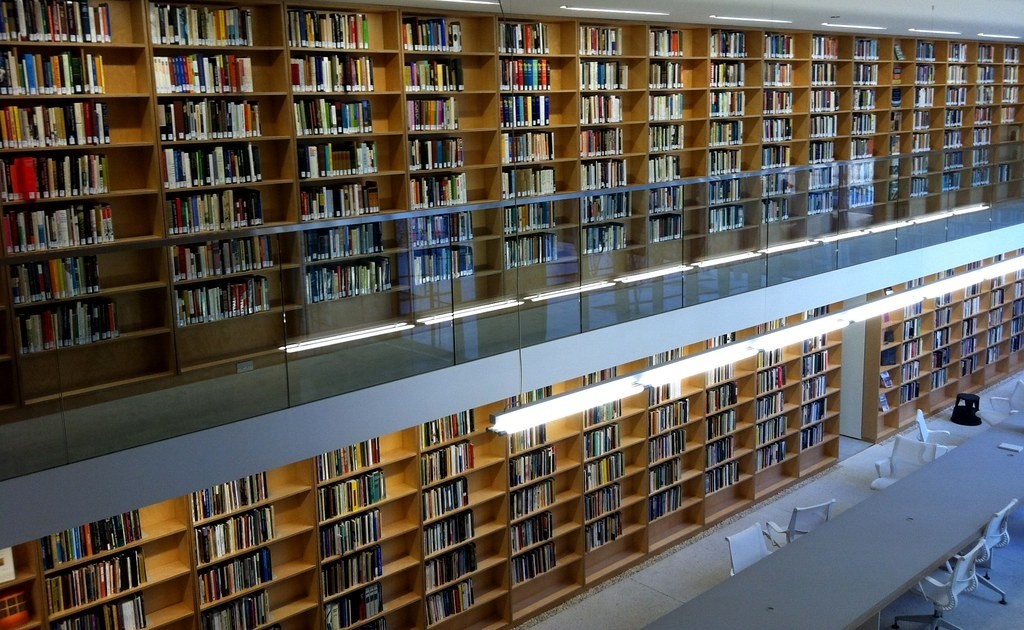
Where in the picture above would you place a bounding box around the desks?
[639,411,1024,630]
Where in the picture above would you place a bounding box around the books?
[0,1,1023,366]
[0,250,1024,630]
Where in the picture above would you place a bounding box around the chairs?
[871,434,938,491]
[948,497,1019,605]
[766,499,839,549]
[915,408,957,465]
[892,537,986,630]
[951,393,983,426]
[725,522,774,577]
[975,379,1024,428]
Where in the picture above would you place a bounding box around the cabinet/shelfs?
[1,301,845,630]
[862,246,1024,443]
[1,0,1024,429]
